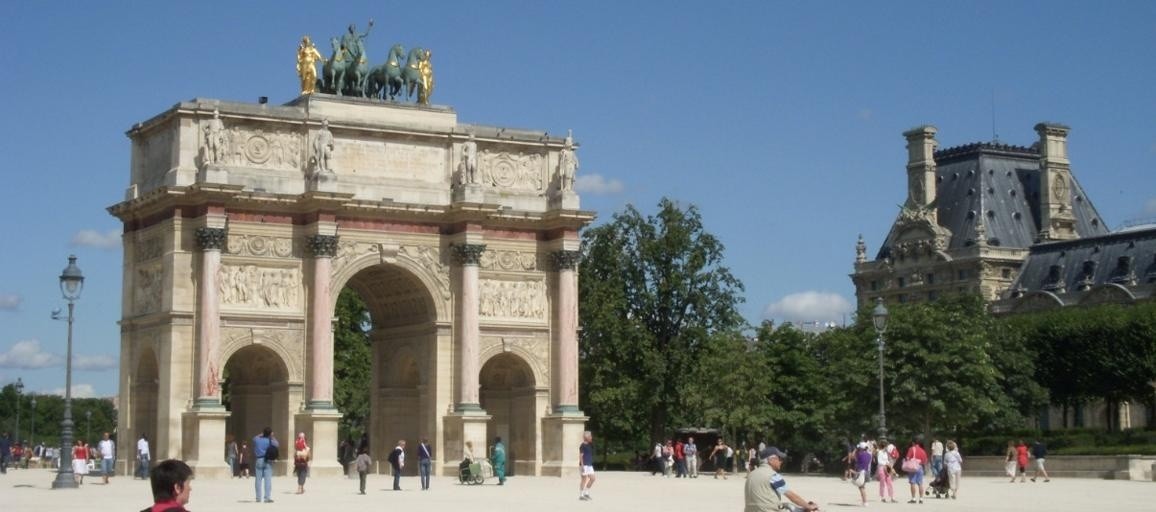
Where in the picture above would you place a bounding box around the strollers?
[925,465,950,499]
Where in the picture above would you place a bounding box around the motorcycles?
[781,500,823,511]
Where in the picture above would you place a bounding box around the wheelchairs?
[459,459,484,484]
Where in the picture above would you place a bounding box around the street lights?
[50,253,85,490]
[12,377,92,461]
[799,320,837,334]
[873,296,889,448]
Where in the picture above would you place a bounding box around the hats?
[759,446,785,458]
[857,442,867,450]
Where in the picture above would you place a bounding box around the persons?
[136,432,151,480]
[477,279,545,318]
[743,446,821,512]
[458,134,579,190]
[648,436,823,481]
[417,436,432,491]
[239,440,250,479]
[294,456,309,495]
[355,448,373,494]
[1030,438,1050,482]
[388,440,406,490]
[252,426,280,503]
[1016,439,1030,482]
[458,442,474,481]
[398,245,450,293]
[217,264,298,308]
[0,430,117,487]
[140,458,195,512]
[419,50,433,105]
[200,108,334,172]
[340,18,372,58]
[295,433,310,460]
[839,432,965,508]
[491,436,507,485]
[226,437,238,475]
[330,241,368,276]
[578,430,597,500]
[1003,440,1018,482]
[296,36,323,95]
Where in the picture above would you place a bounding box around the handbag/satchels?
[851,470,864,486]
[901,457,921,472]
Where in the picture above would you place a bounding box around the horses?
[348,40,368,98]
[322,37,348,96]
[389,47,425,101]
[368,44,406,100]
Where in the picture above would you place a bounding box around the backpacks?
[264,439,279,463]
